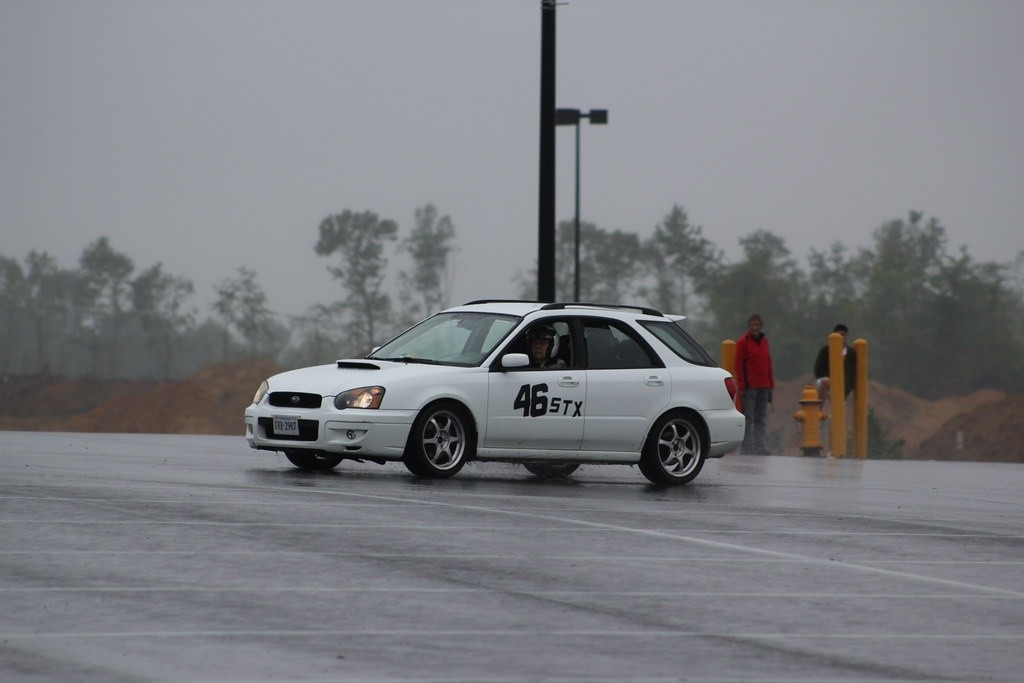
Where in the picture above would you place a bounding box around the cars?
[246,297,748,485]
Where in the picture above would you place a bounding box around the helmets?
[525,325,559,358]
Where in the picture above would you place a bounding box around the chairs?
[557,334,572,369]
[619,340,640,367]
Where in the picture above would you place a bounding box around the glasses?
[840,333,847,336]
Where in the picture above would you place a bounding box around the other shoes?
[827,451,835,459]
[741,447,771,455]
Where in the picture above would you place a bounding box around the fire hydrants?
[793,384,829,456]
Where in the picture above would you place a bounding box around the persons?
[521,324,564,370]
[815,324,856,459]
[736,315,774,455]
